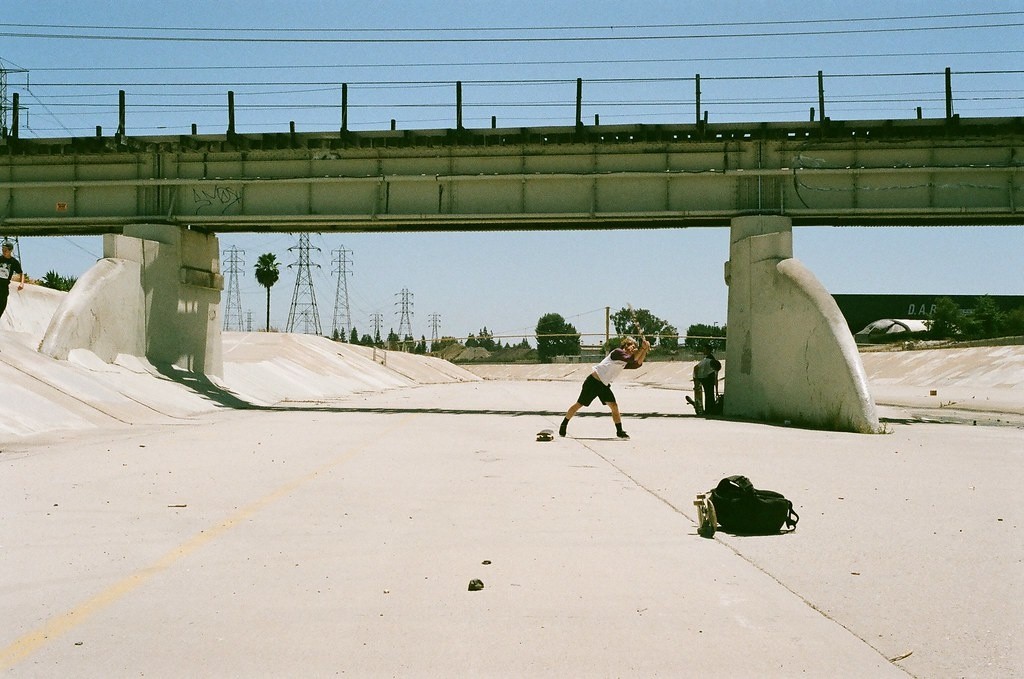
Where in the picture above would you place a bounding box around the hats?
[0,242,13,250]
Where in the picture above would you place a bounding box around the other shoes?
[616,431,630,439]
[559,426,566,437]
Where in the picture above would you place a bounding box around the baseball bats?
[625,300,653,345]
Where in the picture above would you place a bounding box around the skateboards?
[536,429,556,442]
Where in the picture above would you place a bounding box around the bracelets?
[642,347,646,350]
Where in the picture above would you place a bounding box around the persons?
[0,241,24,319]
[692,344,722,416]
[559,336,650,439]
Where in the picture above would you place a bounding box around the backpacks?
[707,476,800,537]
